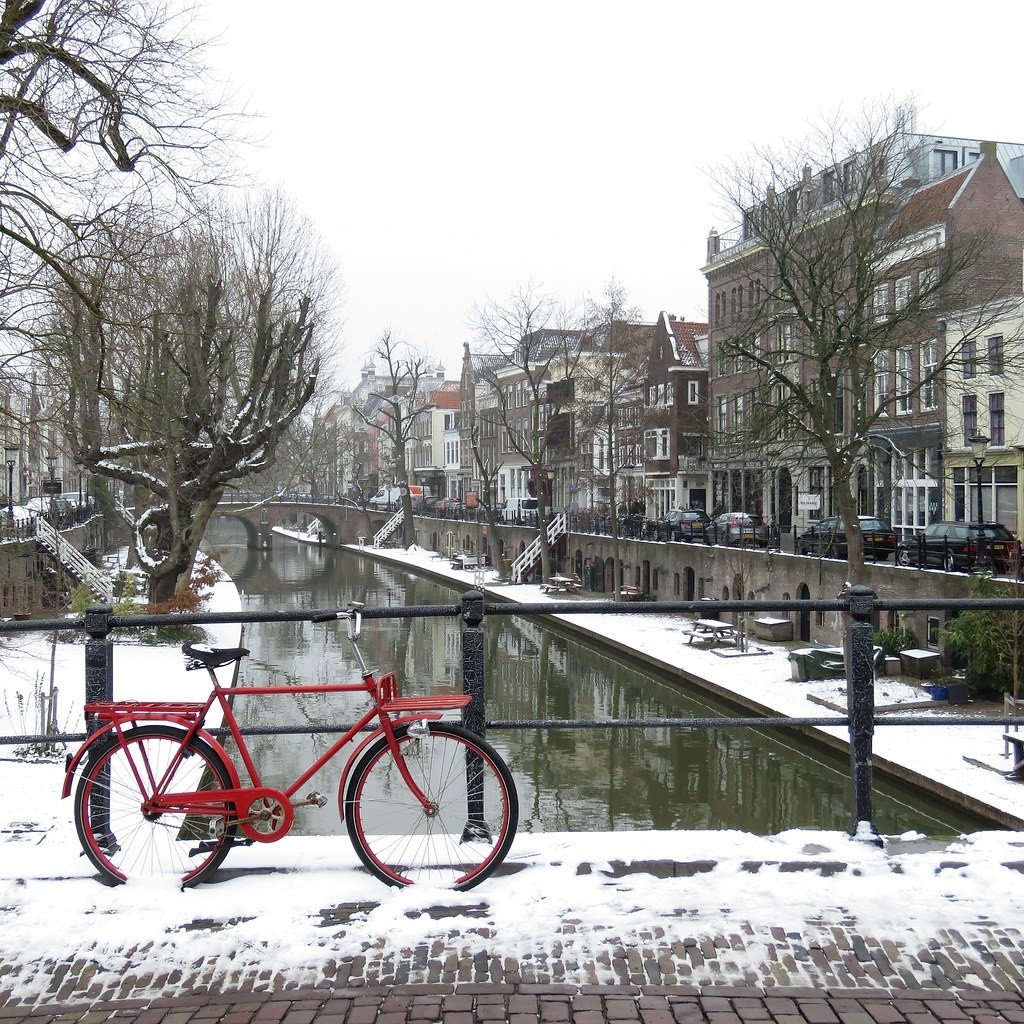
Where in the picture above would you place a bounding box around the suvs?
[897,519,1022,574]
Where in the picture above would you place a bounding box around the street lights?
[622,464,635,538]
[84,467,91,508]
[420,475,427,500]
[765,450,780,554]
[967,428,993,575]
[3,445,19,529]
[76,460,85,506]
[45,455,59,521]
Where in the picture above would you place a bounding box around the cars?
[796,513,895,563]
[704,512,773,549]
[394,495,543,527]
[593,513,655,539]
[650,507,712,544]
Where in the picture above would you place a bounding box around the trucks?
[369,483,432,512]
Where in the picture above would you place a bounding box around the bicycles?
[61,600,521,896]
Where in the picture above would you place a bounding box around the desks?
[451,557,474,570]
[545,576,579,596]
[688,620,738,648]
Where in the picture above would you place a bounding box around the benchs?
[682,630,716,640]
[449,562,460,565]
[541,583,583,589]
[722,630,745,637]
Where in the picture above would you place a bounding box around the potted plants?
[13,596,32,621]
[920,659,955,700]
